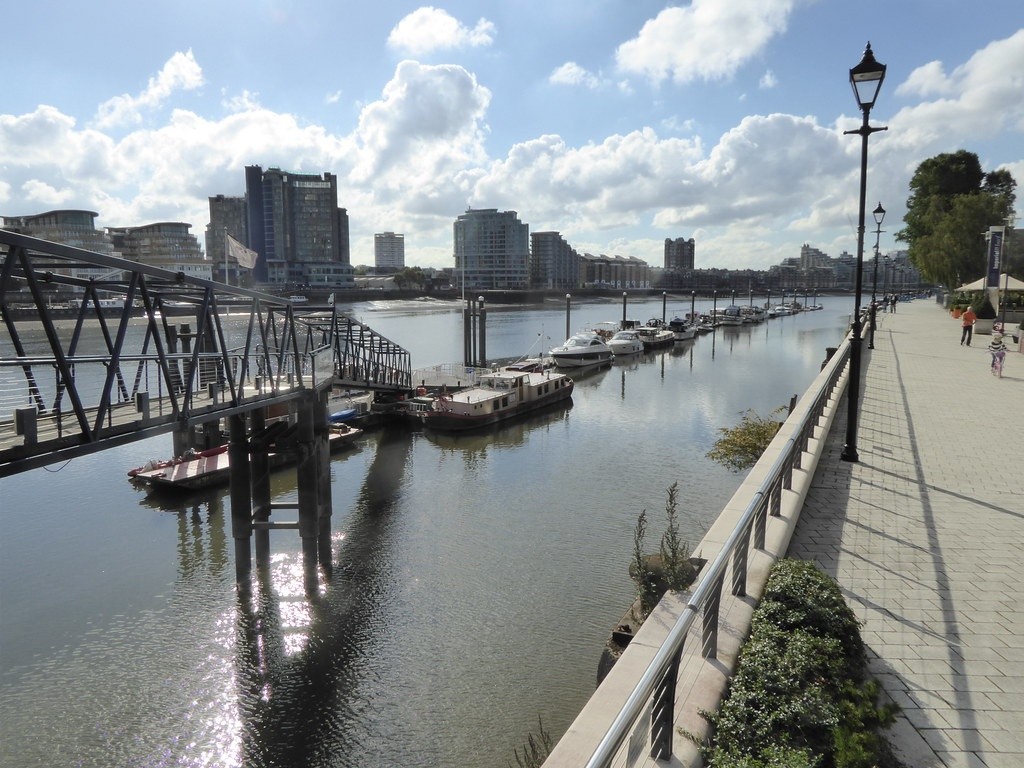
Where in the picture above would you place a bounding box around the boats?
[1,288,313,320]
[423,369,575,433]
[545,300,827,366]
[129,420,364,493]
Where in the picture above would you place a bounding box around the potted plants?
[952,304,960,318]
[1018,318,1024,354]
[972,293,997,336]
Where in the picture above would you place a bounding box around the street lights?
[867,202,886,350]
[871,242,879,304]
[882,254,919,313]
[838,40,892,463]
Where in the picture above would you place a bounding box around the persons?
[988,333,1010,372]
[890,293,898,313]
[960,307,977,346]
[882,295,888,313]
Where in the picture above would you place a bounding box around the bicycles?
[988,347,1007,377]
